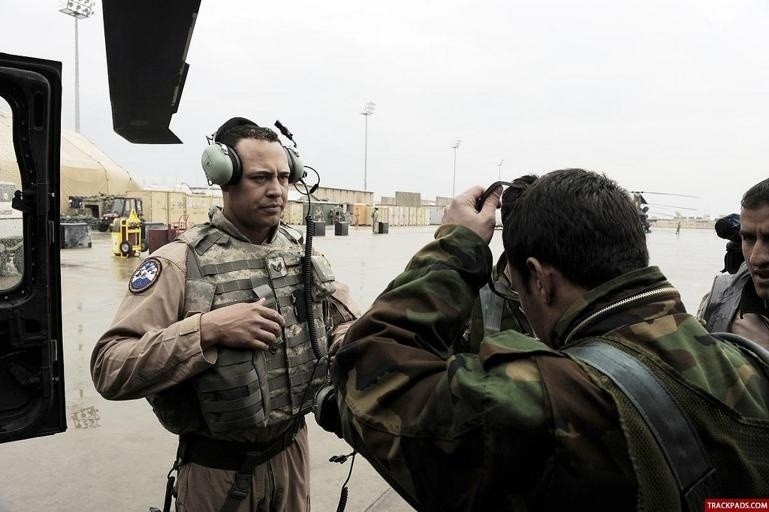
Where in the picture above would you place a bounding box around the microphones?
[330,451,360,464]
[274,120,297,148]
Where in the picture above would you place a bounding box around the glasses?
[489,249,521,302]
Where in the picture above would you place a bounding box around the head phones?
[202,117,305,191]
[313,354,346,438]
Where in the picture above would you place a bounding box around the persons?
[314,207,324,221]
[339,203,345,222]
[698,176,769,353]
[675,221,681,238]
[331,168,768,511]
[92,125,363,512]
[370,208,379,234]
[456,176,542,341]
[354,207,359,229]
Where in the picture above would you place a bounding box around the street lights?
[444,133,463,200]
[59,0,100,137]
[355,100,379,191]
